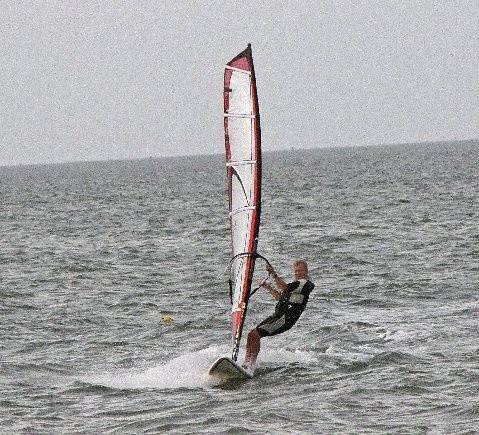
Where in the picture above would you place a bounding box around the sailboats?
[205,42,265,383]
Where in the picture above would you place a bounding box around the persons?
[242,260,315,370]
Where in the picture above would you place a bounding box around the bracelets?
[265,285,272,292]
[272,274,277,279]
